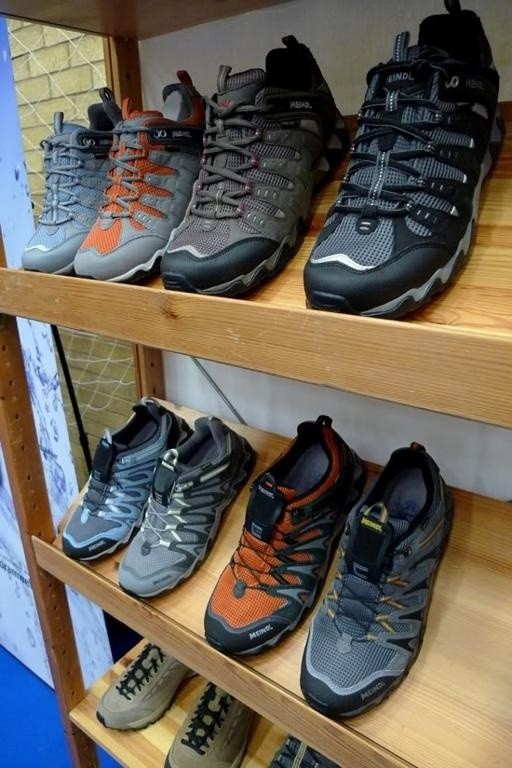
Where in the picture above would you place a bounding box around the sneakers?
[296,435,456,719]
[96,640,199,732]
[19,86,121,277]
[159,32,352,300]
[117,413,258,599]
[202,413,370,658]
[60,395,195,562]
[268,734,340,768]
[72,68,207,286]
[299,0,507,320]
[163,678,256,768]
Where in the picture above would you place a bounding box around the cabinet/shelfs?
[0,0,512,768]
